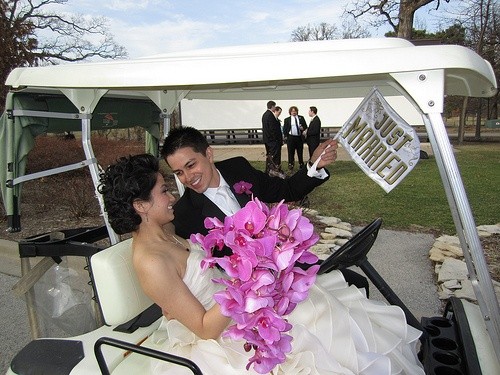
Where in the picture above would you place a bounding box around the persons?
[261,100,283,165]
[160,124,370,300]
[283,106,308,169]
[96,152,427,374]
[302,106,323,158]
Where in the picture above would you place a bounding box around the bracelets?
[308,158,322,171]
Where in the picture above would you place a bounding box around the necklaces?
[169,234,189,252]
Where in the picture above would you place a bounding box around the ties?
[295,117,299,136]
[216,185,241,214]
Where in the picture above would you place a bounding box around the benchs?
[91,237,204,375]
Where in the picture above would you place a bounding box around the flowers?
[190,180,320,375]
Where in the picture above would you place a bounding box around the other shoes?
[300,163,304,168]
[288,163,293,169]
